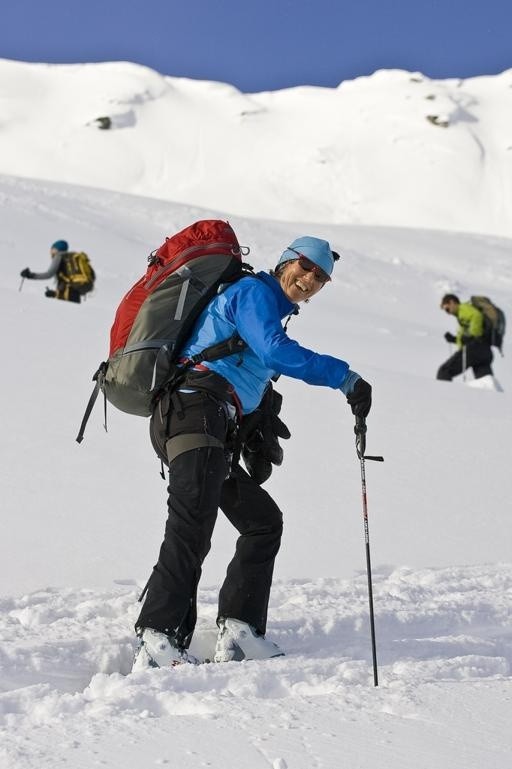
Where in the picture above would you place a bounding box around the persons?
[21,240,81,303]
[133,236,370,673]
[437,294,496,382]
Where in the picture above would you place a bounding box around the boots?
[215,618,281,660]
[133,627,198,671]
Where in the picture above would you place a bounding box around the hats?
[53,240,68,252]
[280,236,335,284]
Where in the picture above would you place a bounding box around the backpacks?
[61,251,94,295]
[76,219,244,443]
[467,297,505,353]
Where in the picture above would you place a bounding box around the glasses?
[298,255,327,284]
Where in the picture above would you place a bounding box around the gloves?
[45,291,57,297]
[461,334,473,344]
[21,267,34,278]
[259,414,290,463]
[445,332,456,343]
[346,377,371,420]
